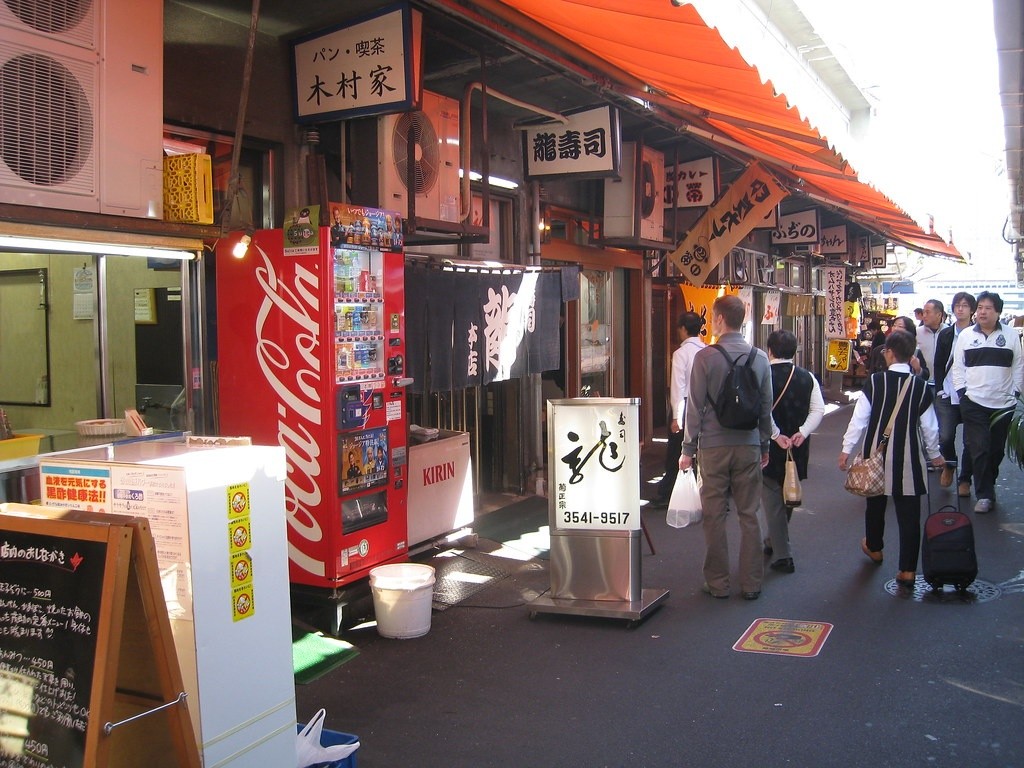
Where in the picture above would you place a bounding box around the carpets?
[291,613,361,685]
[471,495,550,560]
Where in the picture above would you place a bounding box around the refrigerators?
[408,429,475,549]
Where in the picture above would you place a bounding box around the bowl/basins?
[73,417,126,435]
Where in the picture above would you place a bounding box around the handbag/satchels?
[844,442,885,498]
[782,446,803,508]
[665,465,704,529]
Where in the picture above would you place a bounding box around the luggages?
[923,460,977,592]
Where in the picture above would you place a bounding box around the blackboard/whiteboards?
[0,502,200,766]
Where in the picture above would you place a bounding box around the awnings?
[498,0,969,266]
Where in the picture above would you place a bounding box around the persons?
[649,311,707,506]
[761,328,825,574]
[866,321,886,348]
[913,308,924,326]
[679,294,773,599]
[870,316,930,380]
[838,330,945,586]
[952,290,1024,512]
[934,292,979,496]
[916,298,949,472]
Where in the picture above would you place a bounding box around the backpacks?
[704,344,762,431]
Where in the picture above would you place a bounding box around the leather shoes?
[894,570,917,586]
[958,480,972,497]
[862,536,884,564]
[940,465,957,487]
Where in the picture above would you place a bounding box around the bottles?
[346,218,384,247]
[334,252,377,331]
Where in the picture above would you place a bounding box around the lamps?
[764,262,774,272]
[530,201,551,244]
[0,222,204,263]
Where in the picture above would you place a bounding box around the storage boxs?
[163,153,213,224]
[297,723,358,768]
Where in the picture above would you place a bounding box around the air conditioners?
[795,244,820,254]
[0,0,163,221]
[829,238,861,267]
[604,141,665,243]
[351,87,462,224]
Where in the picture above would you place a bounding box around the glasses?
[880,349,888,354]
[953,303,971,308]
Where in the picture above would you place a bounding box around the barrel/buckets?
[369,563,436,639]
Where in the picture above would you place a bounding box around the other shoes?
[702,581,730,599]
[974,498,993,513]
[762,537,773,556]
[740,588,760,600]
[770,557,796,574]
